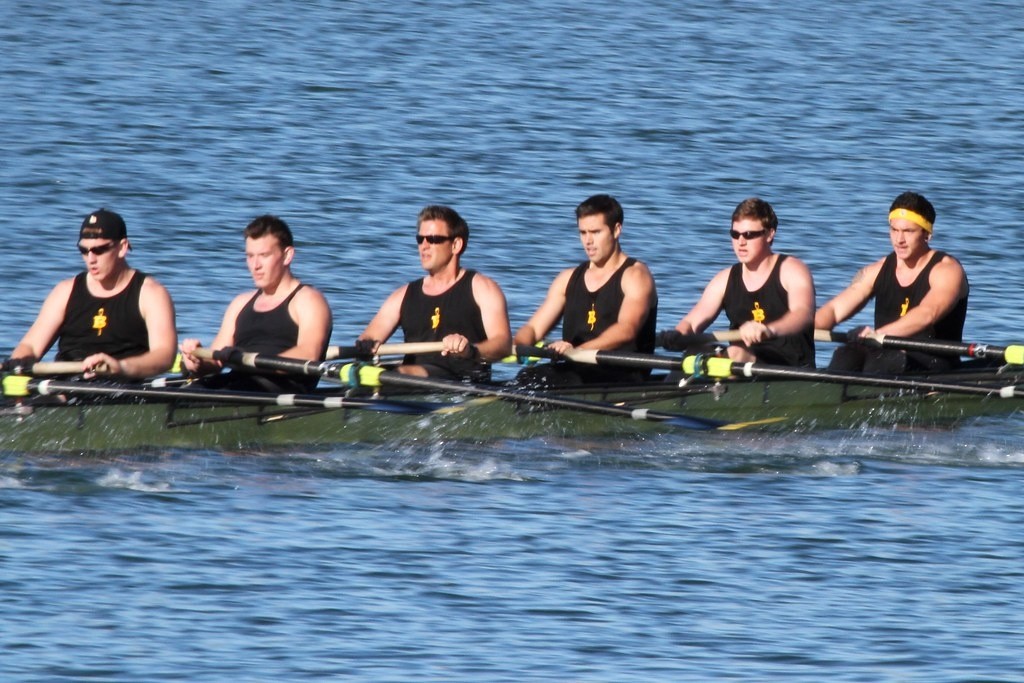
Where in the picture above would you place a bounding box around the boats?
[0,359,1024,468]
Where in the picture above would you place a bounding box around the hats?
[79,209,133,252]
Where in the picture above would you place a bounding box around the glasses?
[415,234,451,244]
[729,229,766,240]
[77,242,116,255]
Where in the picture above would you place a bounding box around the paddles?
[0,326,1024,440]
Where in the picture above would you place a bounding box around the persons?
[175,213,333,395]
[661,196,818,387]
[513,194,658,388]
[814,190,972,378]
[355,205,513,381]
[1,207,178,379]
